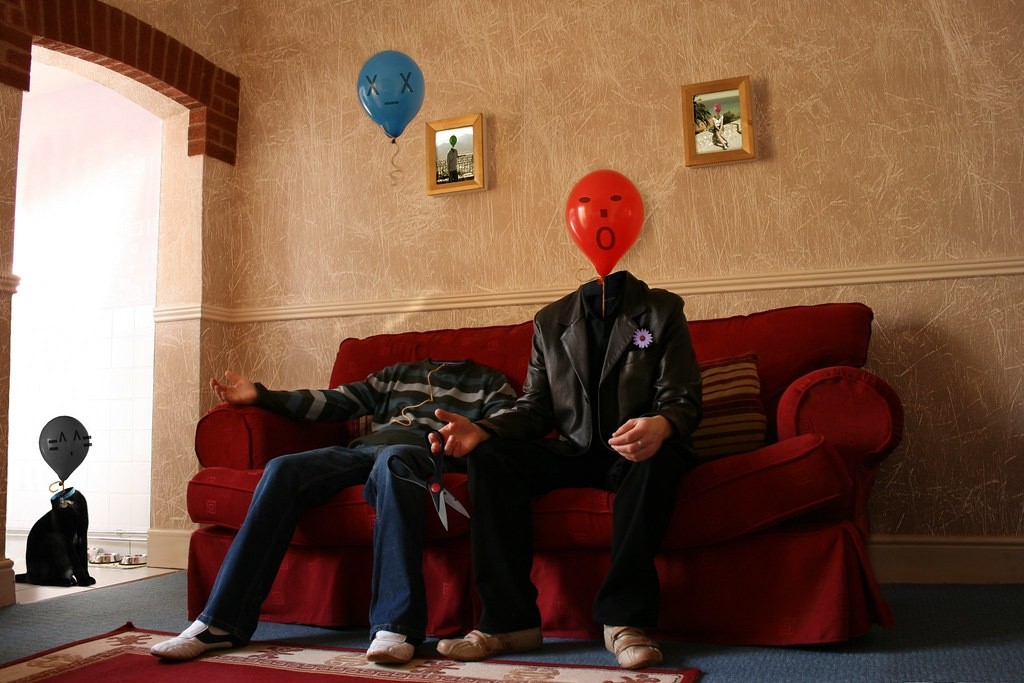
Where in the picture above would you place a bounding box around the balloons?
[449,135,457,147]
[357,49,425,144]
[39,417,93,487]
[564,169,644,285]
[713,104,721,115]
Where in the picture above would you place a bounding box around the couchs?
[185,300,905,649]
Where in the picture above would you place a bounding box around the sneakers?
[365,631,415,663]
[603,624,663,668]
[150,619,233,659]
[436,629,543,660]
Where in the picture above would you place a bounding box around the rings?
[636,440,643,448]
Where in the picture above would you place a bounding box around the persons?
[712,113,729,149]
[149,271,705,672]
[447,148,460,183]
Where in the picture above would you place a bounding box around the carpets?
[0,621,704,683]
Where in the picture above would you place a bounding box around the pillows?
[697,349,771,456]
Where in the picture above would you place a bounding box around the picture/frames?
[425,113,489,197]
[680,74,758,167]
